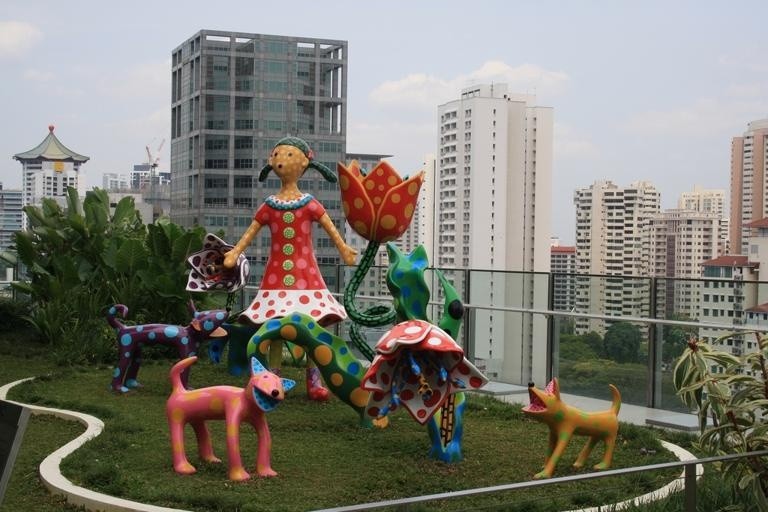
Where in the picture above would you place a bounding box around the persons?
[223,136,357,401]
